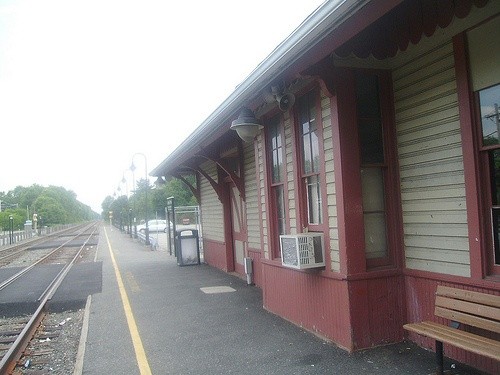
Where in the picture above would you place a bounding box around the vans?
[135,219,174,234]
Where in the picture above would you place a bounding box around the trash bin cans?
[174,228,202,266]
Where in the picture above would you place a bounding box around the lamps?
[230,108,266,144]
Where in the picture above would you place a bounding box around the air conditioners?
[278,232,327,271]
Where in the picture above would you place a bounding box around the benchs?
[403,285,500,375]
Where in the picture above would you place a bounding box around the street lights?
[130,152,151,246]
[10,216,14,244]
[121,171,132,236]
